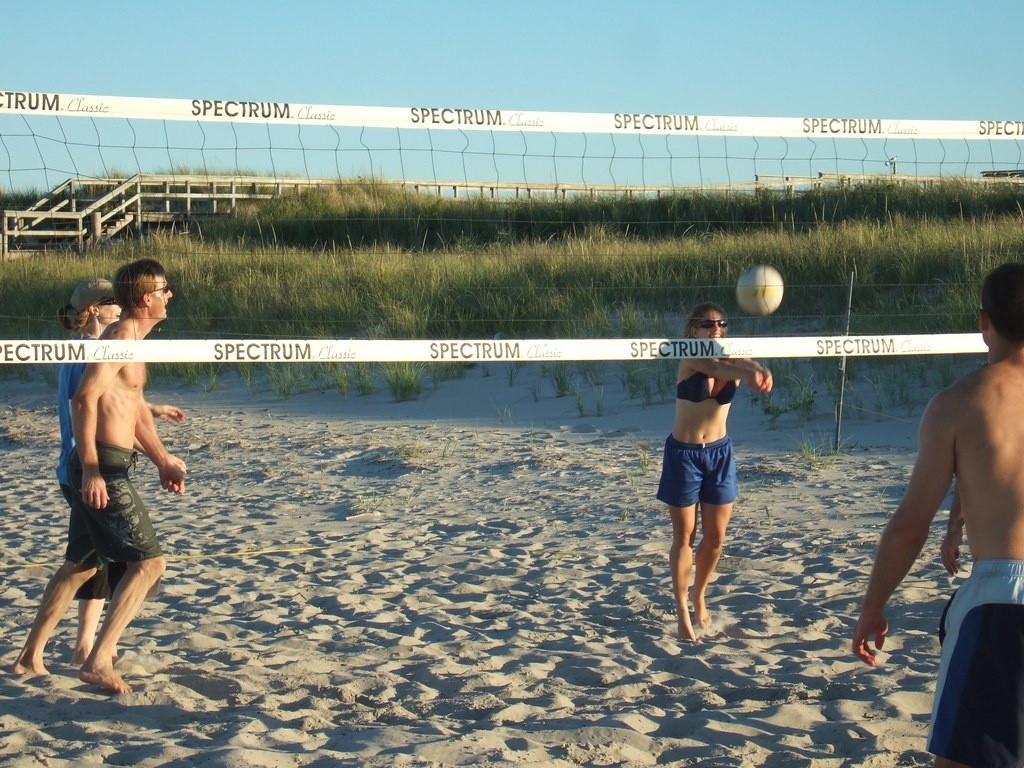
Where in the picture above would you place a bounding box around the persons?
[657,301,774,642]
[851,260,1024,768]
[55,276,186,664]
[11,258,188,695]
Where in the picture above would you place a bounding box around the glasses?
[695,320,728,329]
[142,283,170,298]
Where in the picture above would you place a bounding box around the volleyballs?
[734,264,785,317]
